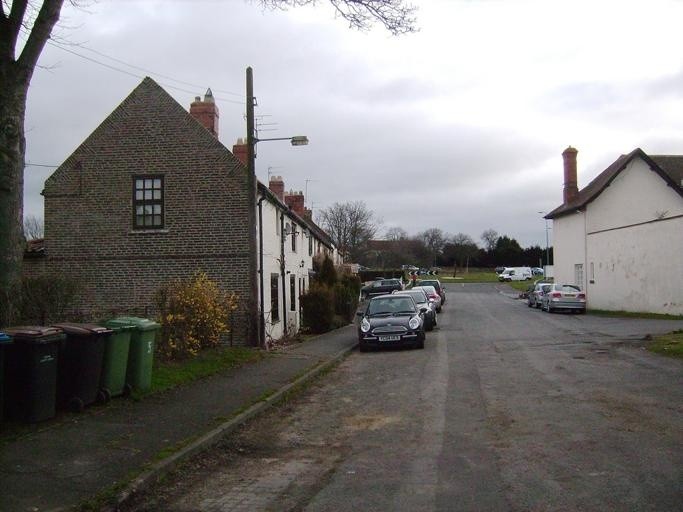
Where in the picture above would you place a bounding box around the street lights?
[244,133,310,349]
[536,210,552,266]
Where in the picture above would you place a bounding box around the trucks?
[496,266,532,282]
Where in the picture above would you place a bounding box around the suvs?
[494,266,507,274]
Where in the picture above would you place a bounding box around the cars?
[349,261,446,333]
[518,265,587,315]
[354,292,428,353]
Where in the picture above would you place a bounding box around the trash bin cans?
[0,316,161,423]
